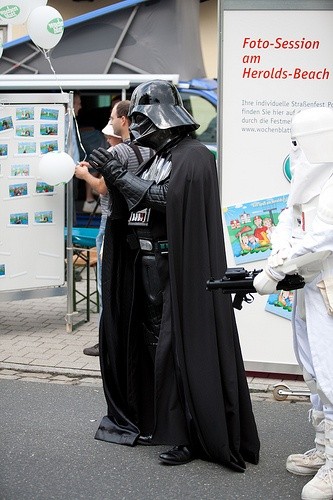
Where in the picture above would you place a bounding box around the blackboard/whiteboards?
[0,104,67,293]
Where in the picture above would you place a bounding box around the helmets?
[290,107,333,164]
[126,80,200,152]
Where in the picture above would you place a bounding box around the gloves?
[88,147,155,212]
[253,269,279,296]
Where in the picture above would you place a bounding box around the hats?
[101,120,122,138]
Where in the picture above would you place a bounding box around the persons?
[65,93,102,212]
[253,107,333,500]
[74,100,156,356]
[88,79,260,474]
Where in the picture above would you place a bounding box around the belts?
[137,236,169,254]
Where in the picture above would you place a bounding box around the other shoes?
[83,343,99,356]
[83,200,101,213]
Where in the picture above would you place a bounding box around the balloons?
[0,0,64,49]
[39,150,75,186]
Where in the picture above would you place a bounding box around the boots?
[286,408,325,475]
[301,419,333,500]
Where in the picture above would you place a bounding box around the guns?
[205,267,305,310]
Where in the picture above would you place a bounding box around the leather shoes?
[159,443,198,464]
[136,435,153,445]
